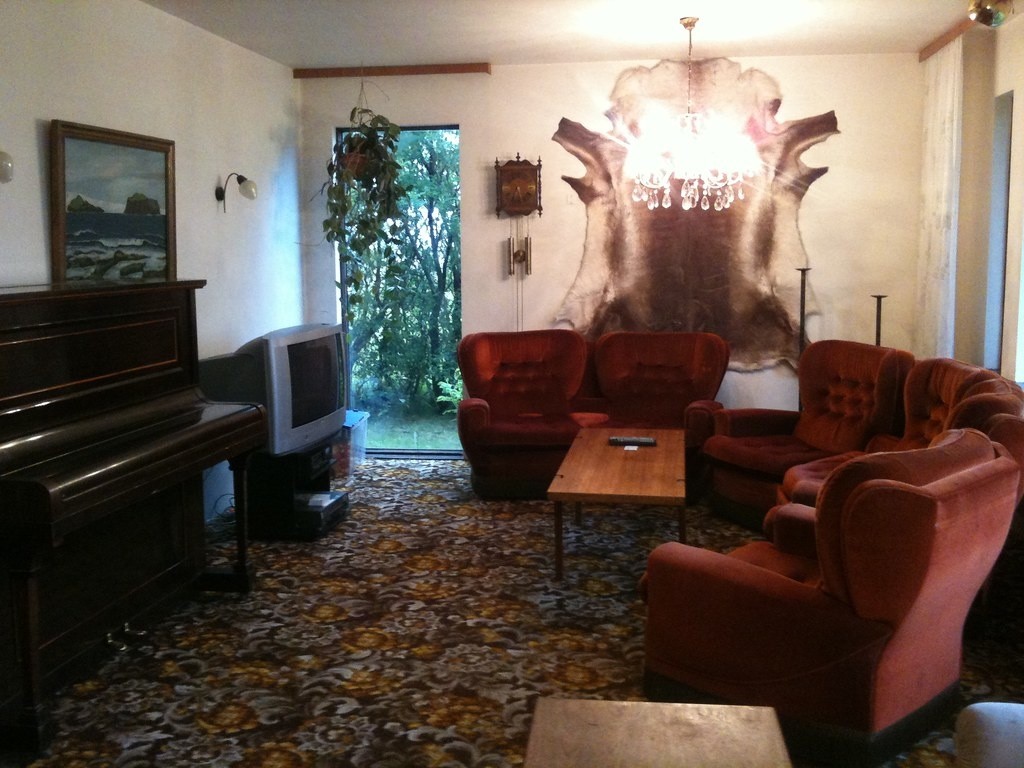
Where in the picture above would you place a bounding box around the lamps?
[966,0,1015,28]
[621,15,763,213]
[215,172,257,213]
[0,151,13,185]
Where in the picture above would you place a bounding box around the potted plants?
[321,61,412,325]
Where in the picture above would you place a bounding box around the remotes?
[609,436,656,447]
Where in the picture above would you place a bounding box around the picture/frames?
[50,119,179,289]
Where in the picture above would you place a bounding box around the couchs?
[637,420,1024,767]
[457,330,587,495]
[779,376,1022,535]
[701,339,917,530]
[582,330,731,466]
[863,354,1004,454]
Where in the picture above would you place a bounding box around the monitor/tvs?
[199,319,347,456]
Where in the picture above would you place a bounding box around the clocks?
[494,151,543,275]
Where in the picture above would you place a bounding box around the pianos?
[0,279,269,755]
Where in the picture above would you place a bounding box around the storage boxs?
[335,410,370,479]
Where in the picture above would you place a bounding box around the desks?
[524,694,791,767]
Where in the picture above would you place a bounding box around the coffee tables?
[543,426,688,581]
